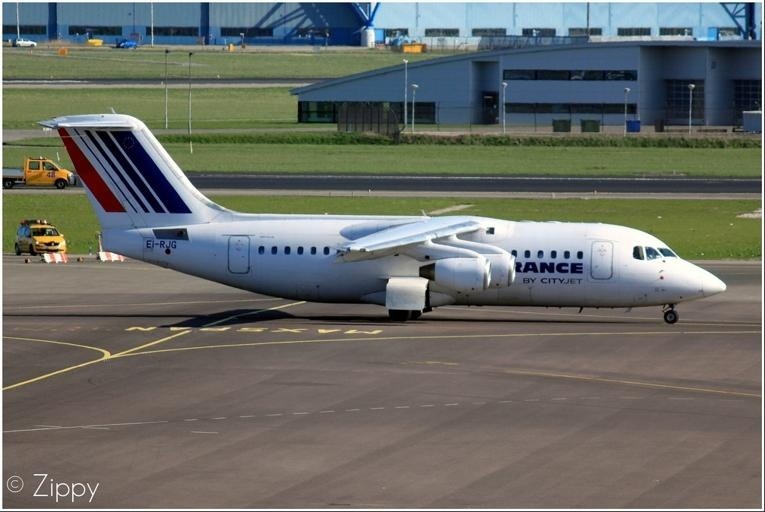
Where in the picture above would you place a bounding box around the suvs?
[14,219,69,255]
[11,38,36,48]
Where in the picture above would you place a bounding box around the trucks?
[4,152,74,190]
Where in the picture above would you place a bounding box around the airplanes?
[38,107,733,325]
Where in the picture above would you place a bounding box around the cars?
[116,39,137,49]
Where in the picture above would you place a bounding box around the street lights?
[188,51,195,131]
[402,58,419,131]
[502,82,508,133]
[623,87,632,138]
[687,82,695,133]
[164,50,171,129]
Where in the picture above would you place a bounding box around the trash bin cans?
[552,119,571,132]
[581,120,600,132]
[627,120,640,132]
[743,111,762,132]
[655,119,664,131]
